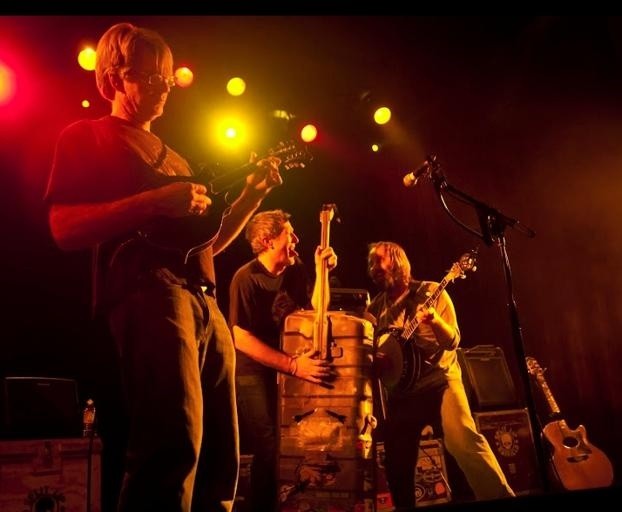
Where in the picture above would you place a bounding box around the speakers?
[457,346,519,409]
[0,374,84,443]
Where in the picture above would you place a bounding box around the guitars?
[139,138,314,254]
[522,356,612,491]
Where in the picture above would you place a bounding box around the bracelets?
[287,356,296,375]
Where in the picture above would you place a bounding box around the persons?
[226,209,340,512]
[362,241,518,511]
[43,22,283,512]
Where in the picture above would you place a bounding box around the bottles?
[83,399,98,437]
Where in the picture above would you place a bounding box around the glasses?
[132,72,177,87]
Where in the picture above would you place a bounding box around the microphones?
[403,161,431,187]
